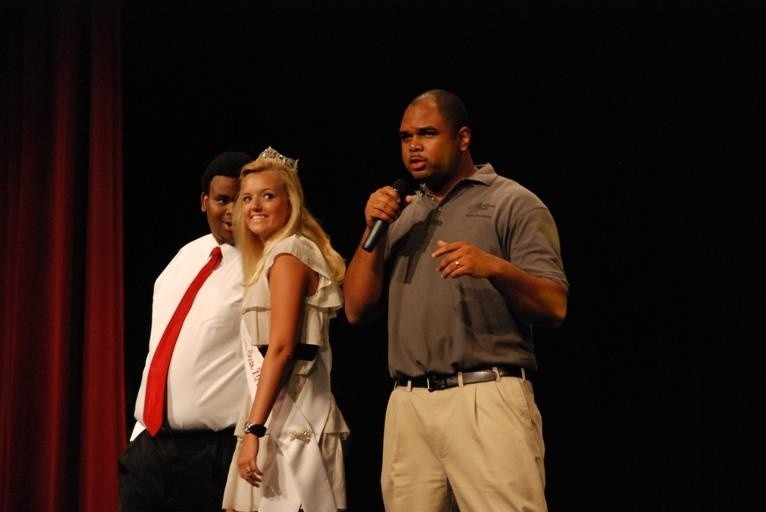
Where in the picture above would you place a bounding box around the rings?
[245,466,252,472]
[453,258,461,270]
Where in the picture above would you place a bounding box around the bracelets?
[239,422,268,438]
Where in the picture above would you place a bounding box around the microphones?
[362,178,407,252]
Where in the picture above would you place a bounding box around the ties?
[143,247,223,438]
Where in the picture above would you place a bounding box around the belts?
[395,365,535,390]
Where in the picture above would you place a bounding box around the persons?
[115,150,265,511]
[216,144,348,512]
[335,82,572,510]
